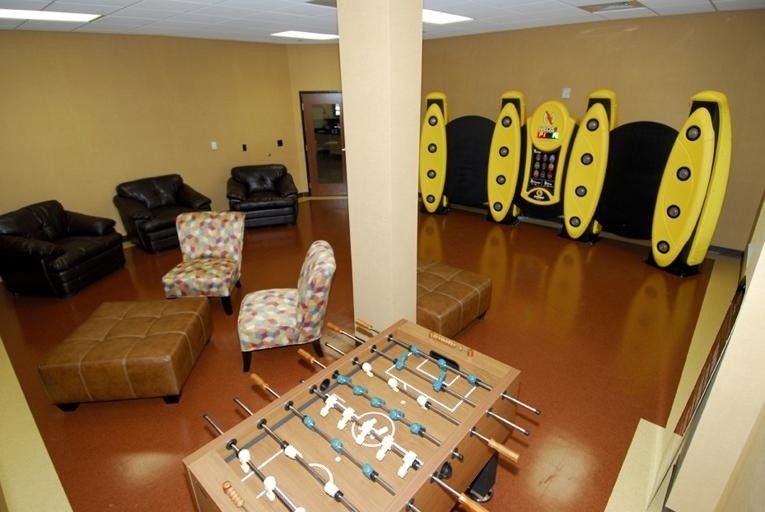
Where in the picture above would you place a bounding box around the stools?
[417,256,492,338]
[35,296,213,412]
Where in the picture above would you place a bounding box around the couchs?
[227,164,299,229]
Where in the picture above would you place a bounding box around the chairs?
[237,240,337,372]
[0,200,127,299]
[113,174,211,255]
[161,211,247,315]
